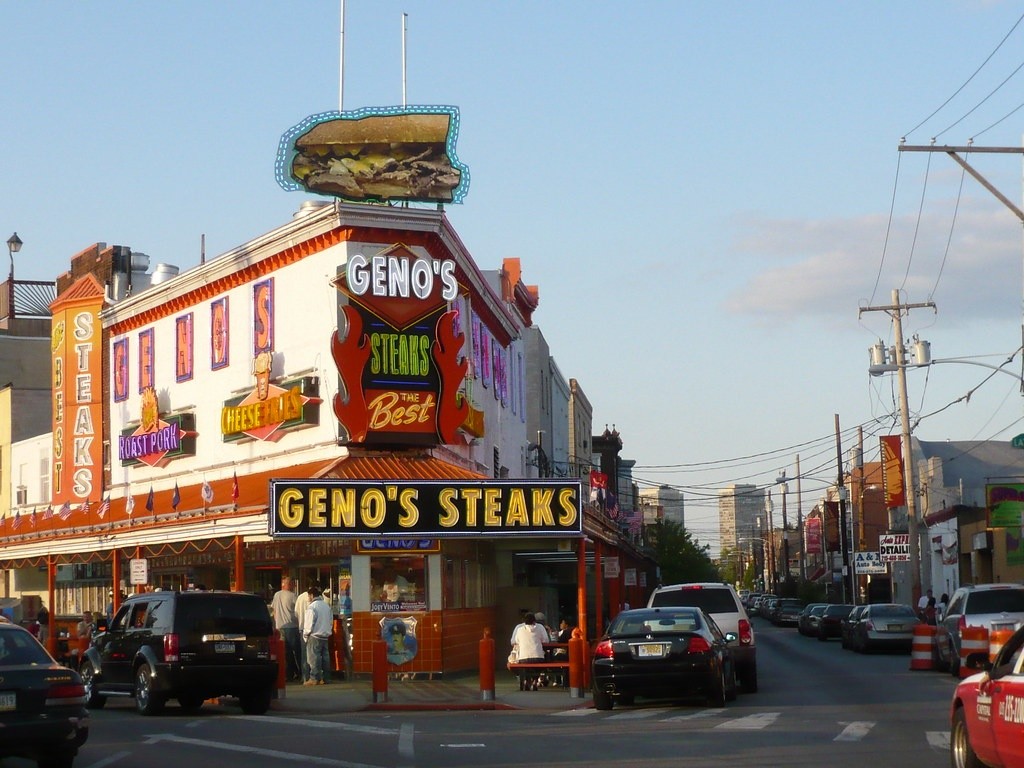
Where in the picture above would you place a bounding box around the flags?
[591,470,643,535]
[0,470,239,531]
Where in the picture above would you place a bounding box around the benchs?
[510,662,570,692]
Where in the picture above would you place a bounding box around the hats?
[534,612,544,622]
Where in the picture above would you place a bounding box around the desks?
[512,643,568,662]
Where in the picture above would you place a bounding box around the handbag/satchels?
[508,646,520,664]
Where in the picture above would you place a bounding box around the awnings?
[809,567,832,584]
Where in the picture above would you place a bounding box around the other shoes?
[525,680,531,691]
[532,683,537,691]
[303,678,317,685]
[552,680,562,687]
[317,680,324,685]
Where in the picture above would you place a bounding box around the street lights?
[775,475,852,606]
[737,537,777,595]
[859,484,877,605]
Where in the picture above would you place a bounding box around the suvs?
[937,581,1024,676]
[643,579,757,694]
[78,590,279,718]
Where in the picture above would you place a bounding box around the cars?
[737,590,804,627]
[839,605,866,650]
[797,603,820,635]
[591,607,738,711]
[851,603,920,655]
[0,622,93,768]
[817,604,856,642]
[950,624,1024,768]
[805,606,827,637]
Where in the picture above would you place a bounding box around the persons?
[34,597,50,646]
[273,576,346,681]
[78,611,97,648]
[918,589,950,626]
[107,596,127,627]
[303,588,333,685]
[511,612,573,691]
